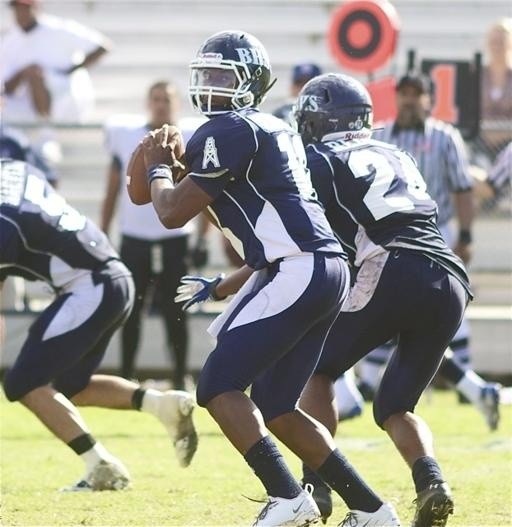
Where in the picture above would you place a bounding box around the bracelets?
[459,227,472,246]
[147,163,174,180]
[210,277,227,302]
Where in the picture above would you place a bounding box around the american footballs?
[125,128,185,206]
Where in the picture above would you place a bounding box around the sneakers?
[411,479,454,527]
[59,457,130,491]
[158,389,198,468]
[339,392,363,420]
[337,501,402,526]
[482,383,499,428]
[252,482,332,526]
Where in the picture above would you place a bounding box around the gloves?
[174,272,223,312]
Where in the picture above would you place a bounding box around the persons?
[96,82,196,390]
[142,31,397,524]
[371,74,476,403]
[326,343,499,426]
[295,72,469,527]
[273,62,320,123]
[476,21,512,122]
[2,2,111,131]
[0,157,197,493]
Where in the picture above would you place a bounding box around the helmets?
[289,73,373,143]
[188,30,271,119]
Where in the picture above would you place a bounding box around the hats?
[293,66,319,82]
[396,72,430,91]
